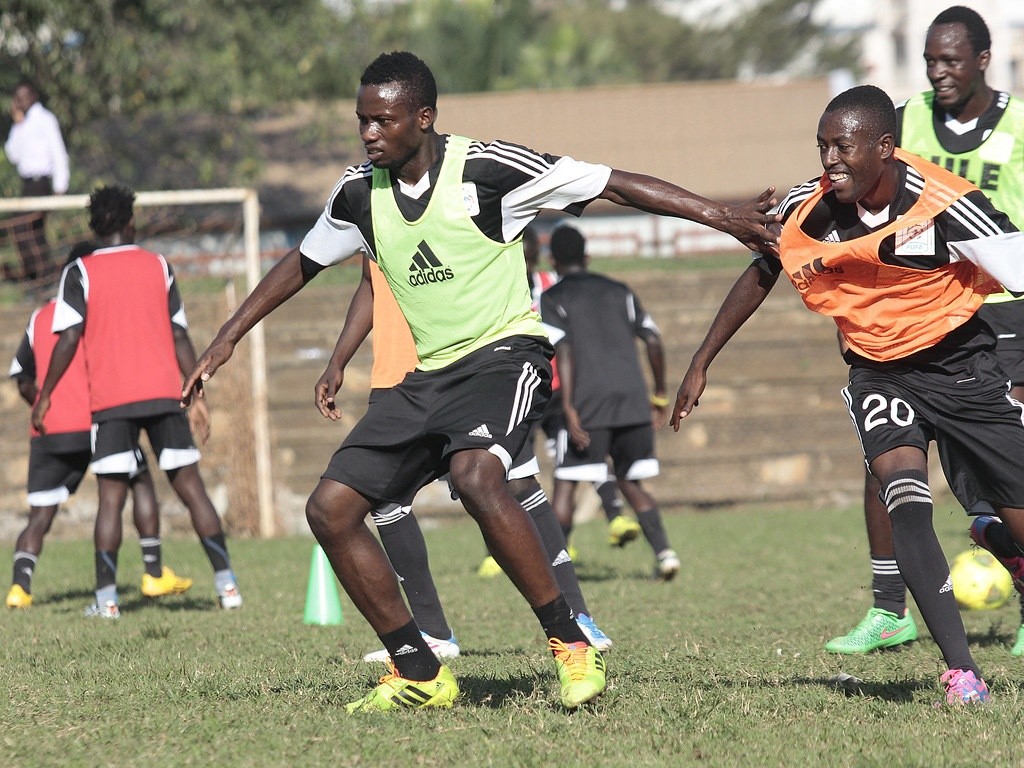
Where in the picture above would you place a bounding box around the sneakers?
[566,545,576,564]
[939,669,989,708]
[825,607,919,655]
[478,555,503,576]
[970,515,1024,595]
[1010,623,1024,659]
[362,626,459,662]
[6,584,32,610]
[656,549,680,577]
[141,566,192,596]
[547,638,606,709]
[608,516,640,547]
[83,602,120,621]
[214,570,242,611]
[346,656,460,717]
[575,613,613,652]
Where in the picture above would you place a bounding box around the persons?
[6,239,194,611]
[666,85,1023,709]
[5,86,71,284]
[30,184,241,619]
[827,5,1021,653]
[179,51,783,720]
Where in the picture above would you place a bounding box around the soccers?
[948,547,1019,613]
